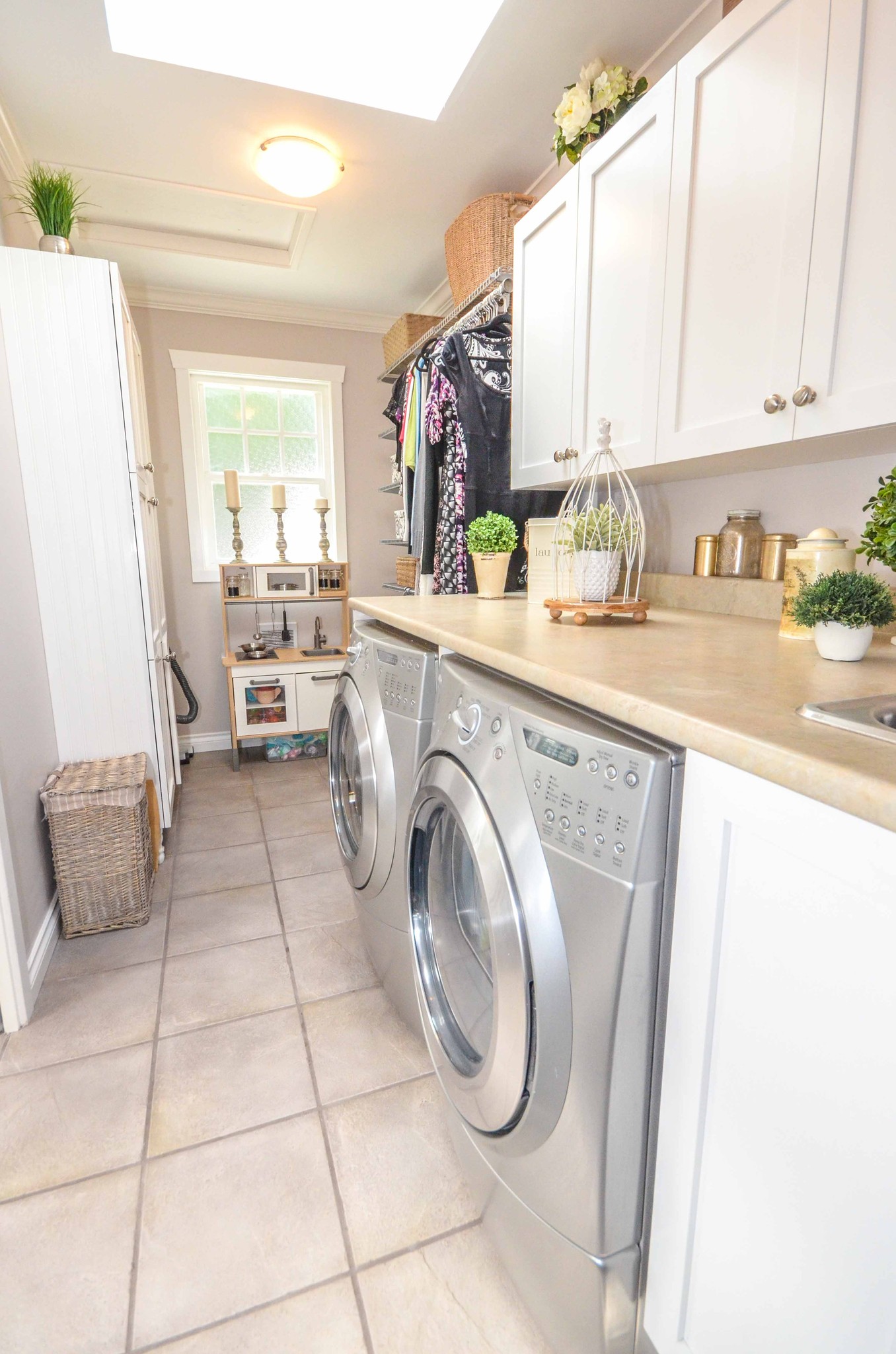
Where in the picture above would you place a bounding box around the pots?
[247,648,275,658]
[271,583,299,590]
[238,643,270,653]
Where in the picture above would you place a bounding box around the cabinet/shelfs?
[377,425,416,595]
[509,63,676,491]
[644,748,895,1350]
[655,1,896,481]
[1,245,182,862]
[221,563,350,772]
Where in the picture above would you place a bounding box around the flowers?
[550,57,649,165]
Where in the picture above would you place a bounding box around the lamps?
[252,136,344,200]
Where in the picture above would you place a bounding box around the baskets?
[394,556,418,589]
[39,752,160,940]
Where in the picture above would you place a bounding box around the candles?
[224,470,241,507]
[272,484,286,507]
[316,499,328,508]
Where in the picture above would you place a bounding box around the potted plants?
[787,566,896,662]
[552,502,640,602]
[463,509,519,600]
[855,465,896,590]
[0,157,102,257]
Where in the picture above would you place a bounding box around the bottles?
[715,509,765,578]
[226,576,241,598]
[329,569,344,591]
[237,574,251,598]
[318,569,329,592]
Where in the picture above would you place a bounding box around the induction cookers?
[235,649,279,662]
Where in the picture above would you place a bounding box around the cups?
[759,533,797,580]
[693,534,719,576]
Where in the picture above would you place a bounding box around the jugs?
[250,686,281,704]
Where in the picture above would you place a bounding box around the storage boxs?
[261,731,328,763]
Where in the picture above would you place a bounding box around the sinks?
[798,693,896,741]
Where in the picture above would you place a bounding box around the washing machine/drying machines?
[401,650,687,1354]
[326,618,440,1042]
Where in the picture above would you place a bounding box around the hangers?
[412,284,513,378]
